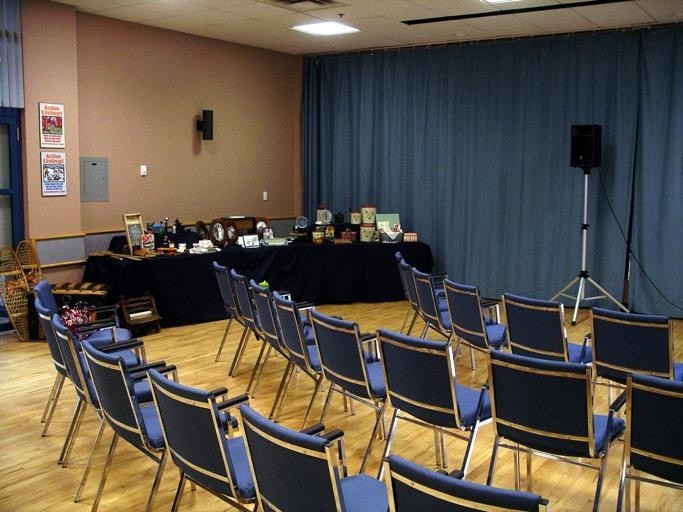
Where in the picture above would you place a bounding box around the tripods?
[549,168,633,326]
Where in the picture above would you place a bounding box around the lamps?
[195,108,215,141]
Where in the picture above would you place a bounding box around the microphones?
[569,122,603,168]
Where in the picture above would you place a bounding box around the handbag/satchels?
[62,302,97,339]
